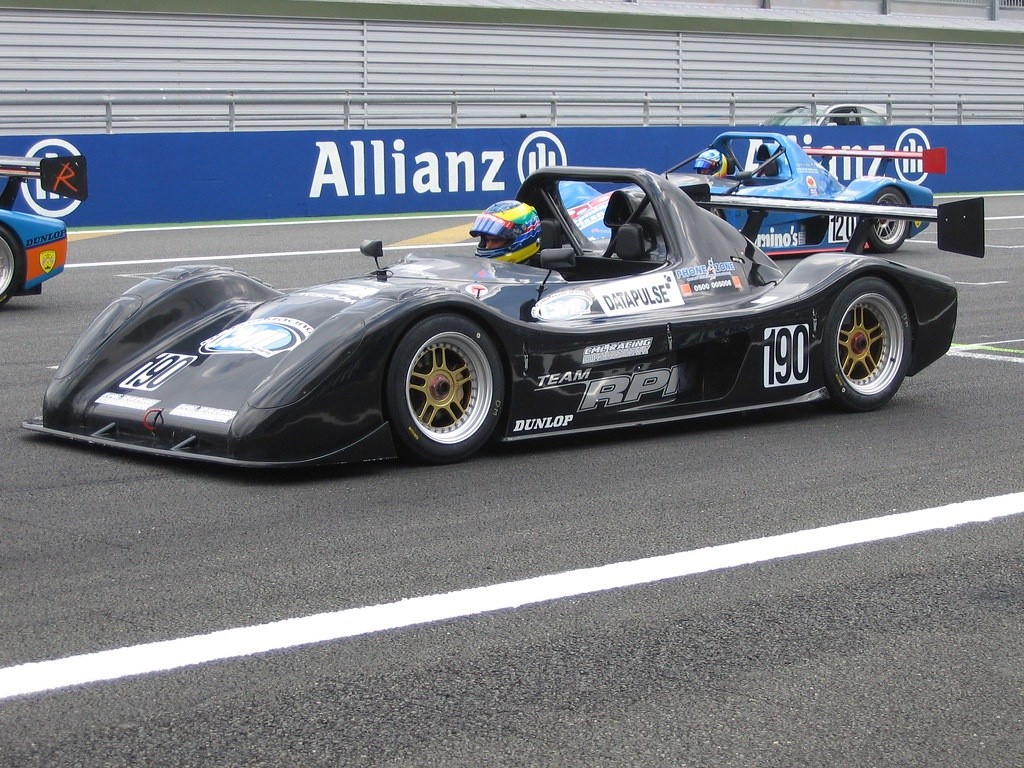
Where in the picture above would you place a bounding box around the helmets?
[693,149,727,178]
[470,200,541,264]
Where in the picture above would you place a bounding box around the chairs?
[523,219,583,282]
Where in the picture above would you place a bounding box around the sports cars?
[0,151,90,309]
[17,151,962,474]
[558,128,938,260]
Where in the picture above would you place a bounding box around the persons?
[694,149,727,178]
[469,200,542,266]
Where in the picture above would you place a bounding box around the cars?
[760,105,886,126]
